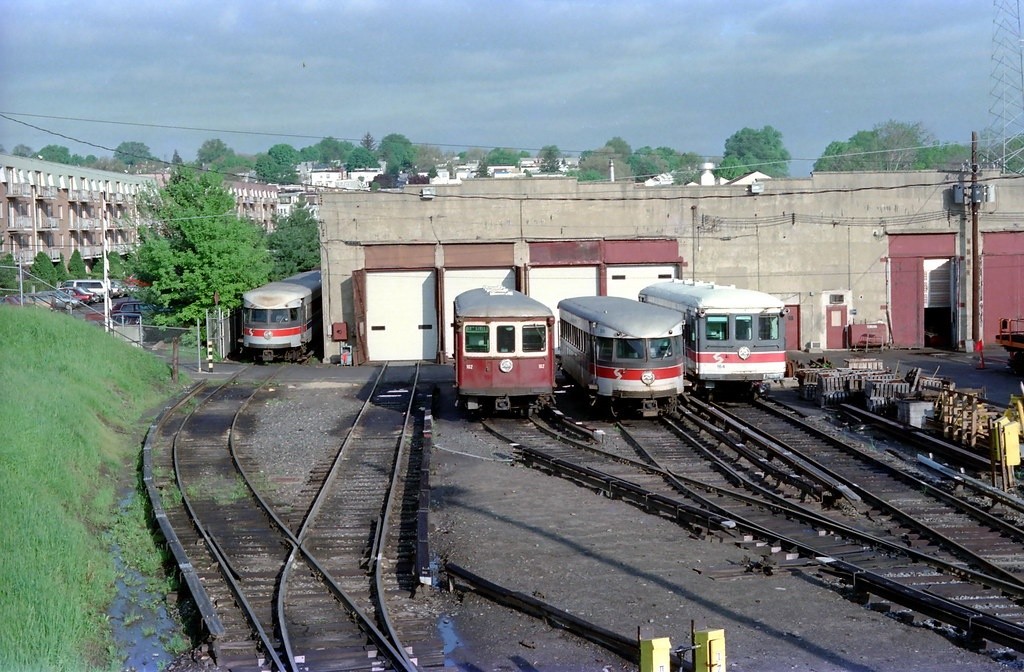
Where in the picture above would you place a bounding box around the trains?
[638,277,787,405]
[558,295,684,418]
[450,285,554,422]
[245,269,322,364]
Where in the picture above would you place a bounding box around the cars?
[59,289,91,305]
[38,291,83,310]
[64,287,97,305]
[86,301,169,326]
[108,279,140,299]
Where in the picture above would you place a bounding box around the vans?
[56,279,105,303]
[125,273,152,287]
